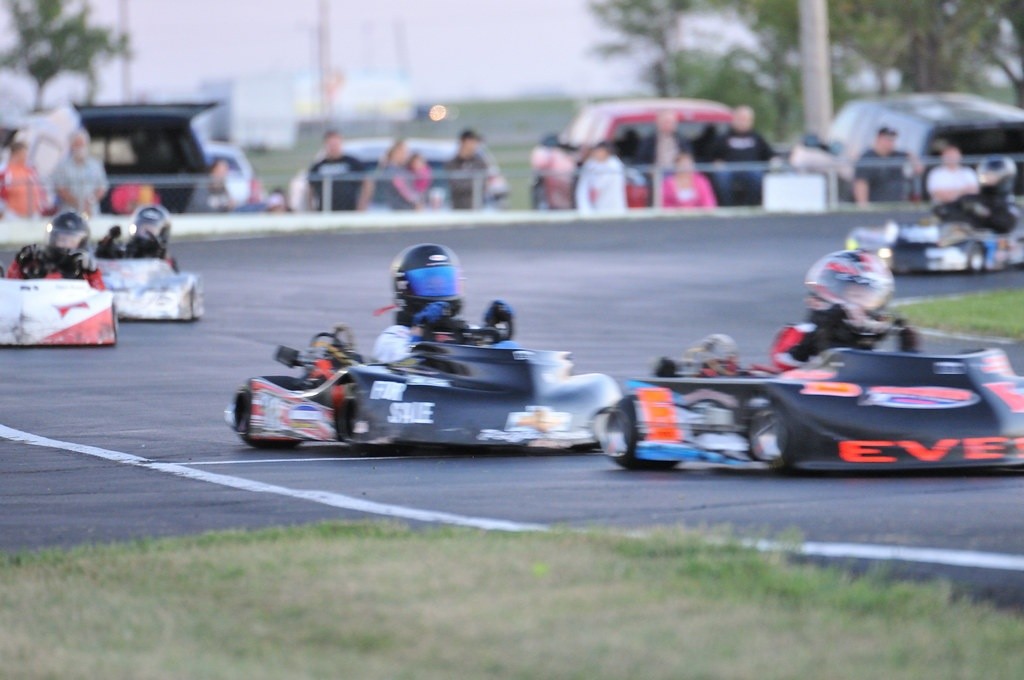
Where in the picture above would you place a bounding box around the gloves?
[799,330,827,355]
[17,243,46,263]
[899,326,923,352]
[485,300,512,326]
[75,252,98,274]
[413,300,451,326]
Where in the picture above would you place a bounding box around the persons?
[932,155,1018,235]
[771,247,916,372]
[0,143,47,218]
[307,130,375,212]
[927,146,980,200]
[209,156,235,212]
[655,105,781,208]
[264,189,292,211]
[7,211,106,290]
[51,129,109,214]
[530,133,629,209]
[378,128,492,211]
[795,126,923,203]
[96,205,171,259]
[370,242,522,366]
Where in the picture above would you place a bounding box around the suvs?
[0,102,257,219]
[827,90,1024,161]
[529,99,732,209]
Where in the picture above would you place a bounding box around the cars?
[294,139,509,210]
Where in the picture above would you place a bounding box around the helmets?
[47,212,88,252]
[391,242,464,304]
[800,250,898,331]
[133,205,171,248]
